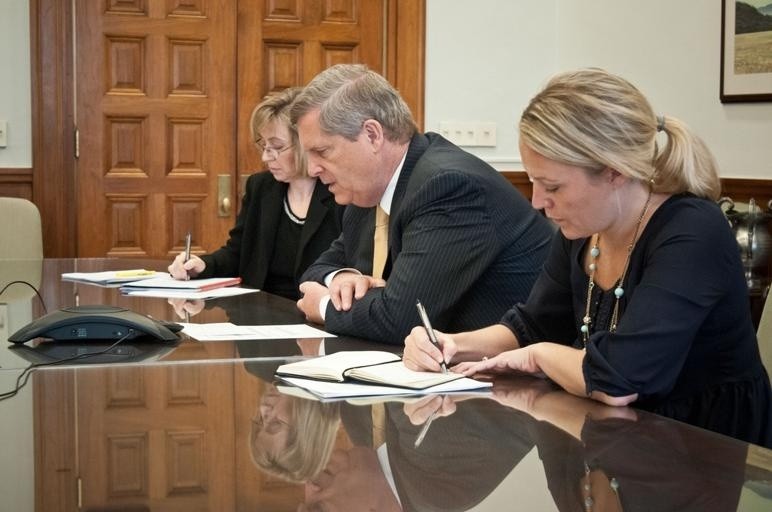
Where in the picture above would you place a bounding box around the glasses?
[253,135,300,158]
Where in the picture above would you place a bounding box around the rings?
[480,353,491,362]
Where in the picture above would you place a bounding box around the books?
[276,344,472,393]
[120,268,242,295]
[60,262,169,287]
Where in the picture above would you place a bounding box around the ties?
[371,204,390,288]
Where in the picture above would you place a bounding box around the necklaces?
[579,447,621,511]
[578,185,658,345]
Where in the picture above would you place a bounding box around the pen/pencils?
[416,298,447,371]
[185,232,192,281]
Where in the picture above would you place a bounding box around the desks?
[0,259,772,511]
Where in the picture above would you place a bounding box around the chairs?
[0,197,43,259]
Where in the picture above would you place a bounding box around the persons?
[522,387,749,510]
[164,290,351,486]
[400,66,771,446]
[295,396,538,512]
[290,58,557,345]
[168,84,347,306]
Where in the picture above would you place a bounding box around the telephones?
[8,306,184,345]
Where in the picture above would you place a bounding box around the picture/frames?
[720,0,772,103]
